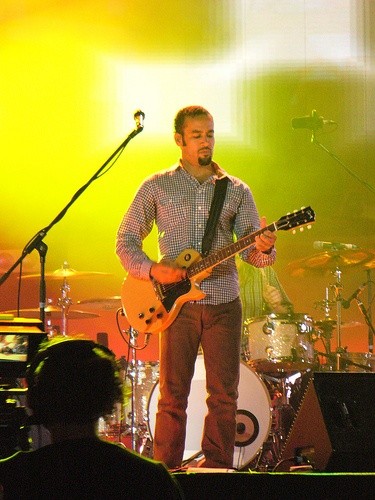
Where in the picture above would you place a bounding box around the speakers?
[276,372,375,473]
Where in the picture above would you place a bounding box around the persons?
[0,336,183,500]
[115,106,276,468]
[234,252,294,362]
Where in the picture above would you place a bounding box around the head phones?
[25,338,121,411]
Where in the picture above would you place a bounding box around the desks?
[0,326,47,449]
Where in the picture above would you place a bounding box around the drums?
[323,350,375,371]
[112,362,158,435]
[147,355,272,471]
[243,312,319,376]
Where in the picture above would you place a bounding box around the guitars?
[120,205,316,334]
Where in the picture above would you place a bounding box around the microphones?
[290,117,335,130]
[133,109,145,132]
[342,284,366,309]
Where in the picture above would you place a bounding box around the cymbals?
[311,321,365,331]
[16,263,115,283]
[285,251,367,277]
[0,297,103,318]
[76,295,120,305]
[312,300,338,306]
